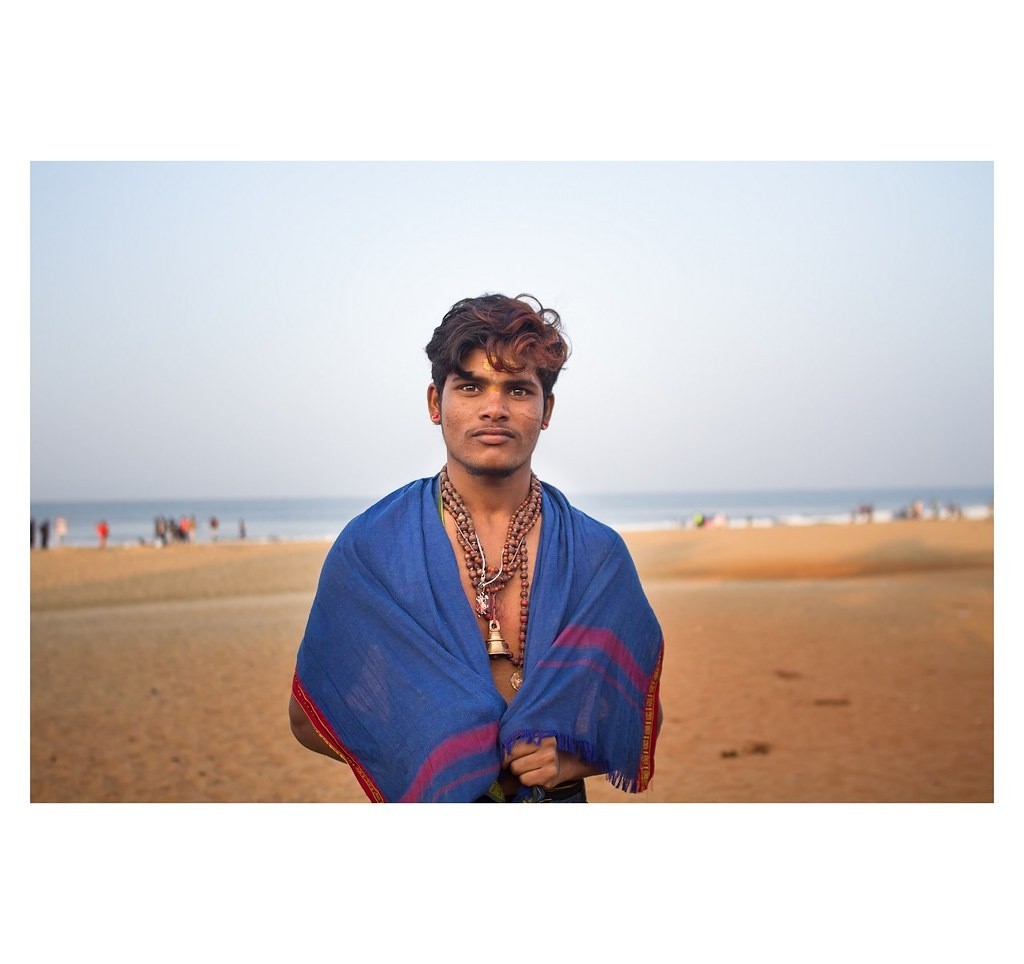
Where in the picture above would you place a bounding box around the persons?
[288,294,664,803]
[30,514,247,549]
[694,499,963,527]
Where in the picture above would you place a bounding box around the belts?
[510,781,582,803]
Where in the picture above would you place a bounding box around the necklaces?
[441,462,542,692]
